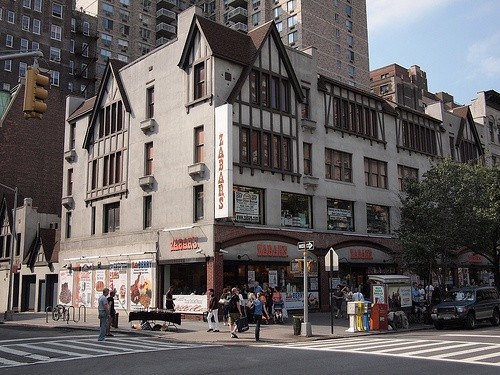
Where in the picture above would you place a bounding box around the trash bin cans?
[346,301,372,333]
[370,303,389,330]
[293,315,304,336]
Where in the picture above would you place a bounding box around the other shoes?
[213,330,219,332]
[231,335,234,338]
[105,334,113,336]
[206,329,213,332]
[231,331,238,338]
[335,314,349,319]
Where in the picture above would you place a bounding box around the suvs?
[430,285,500,330]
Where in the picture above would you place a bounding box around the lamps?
[382,259,397,264]
[339,258,353,262]
[238,253,253,262]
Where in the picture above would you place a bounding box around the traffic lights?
[290,261,302,274]
[307,261,318,275]
[23,69,50,115]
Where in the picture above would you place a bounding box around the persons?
[216,282,286,325]
[97,288,111,341]
[411,281,443,305]
[228,288,241,339]
[104,291,116,336]
[333,281,365,319]
[166,287,175,311]
[465,280,495,300]
[206,288,221,332]
[202,284,208,295]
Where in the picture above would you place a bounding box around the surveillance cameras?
[196,249,203,254]
[219,249,228,254]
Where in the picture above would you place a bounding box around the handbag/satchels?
[218,299,225,304]
[235,317,249,332]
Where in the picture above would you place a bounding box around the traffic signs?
[296,241,314,251]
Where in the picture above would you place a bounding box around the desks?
[128,310,181,332]
